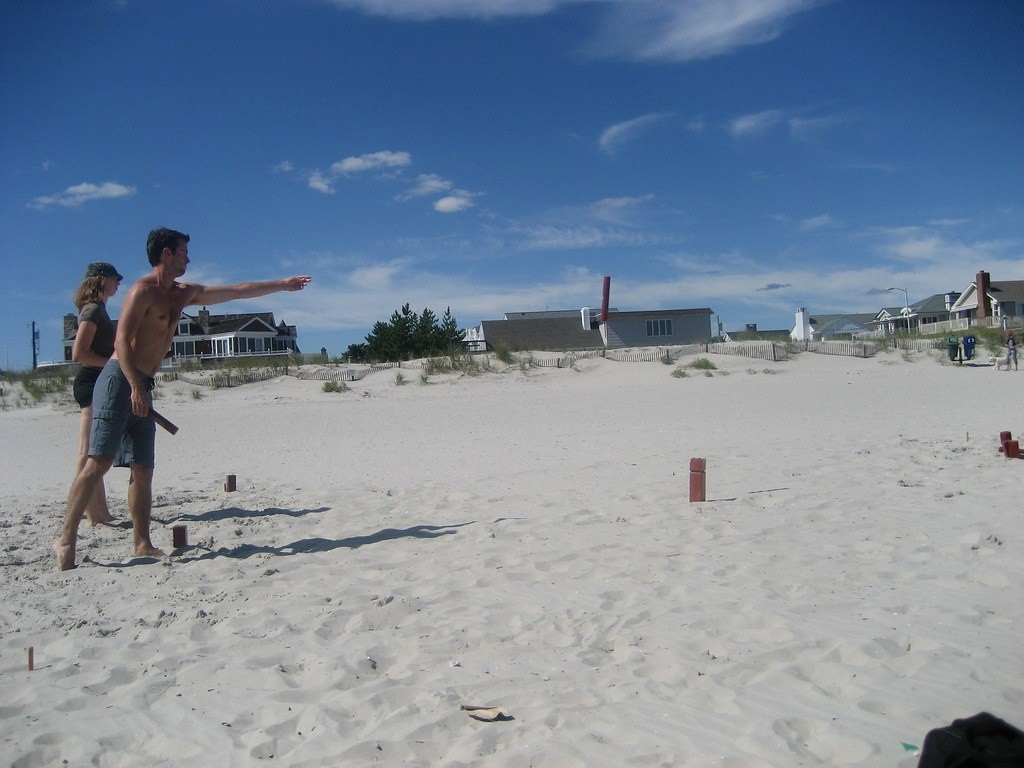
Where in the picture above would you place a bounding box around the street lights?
[886,287,910,336]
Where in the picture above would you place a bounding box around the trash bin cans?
[946,335,976,361]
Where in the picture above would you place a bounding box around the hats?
[85,262,124,281]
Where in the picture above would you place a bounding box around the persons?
[73,263,123,527]
[52,227,312,571]
[1005,332,1017,371]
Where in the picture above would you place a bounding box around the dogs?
[989,357,1011,371]
